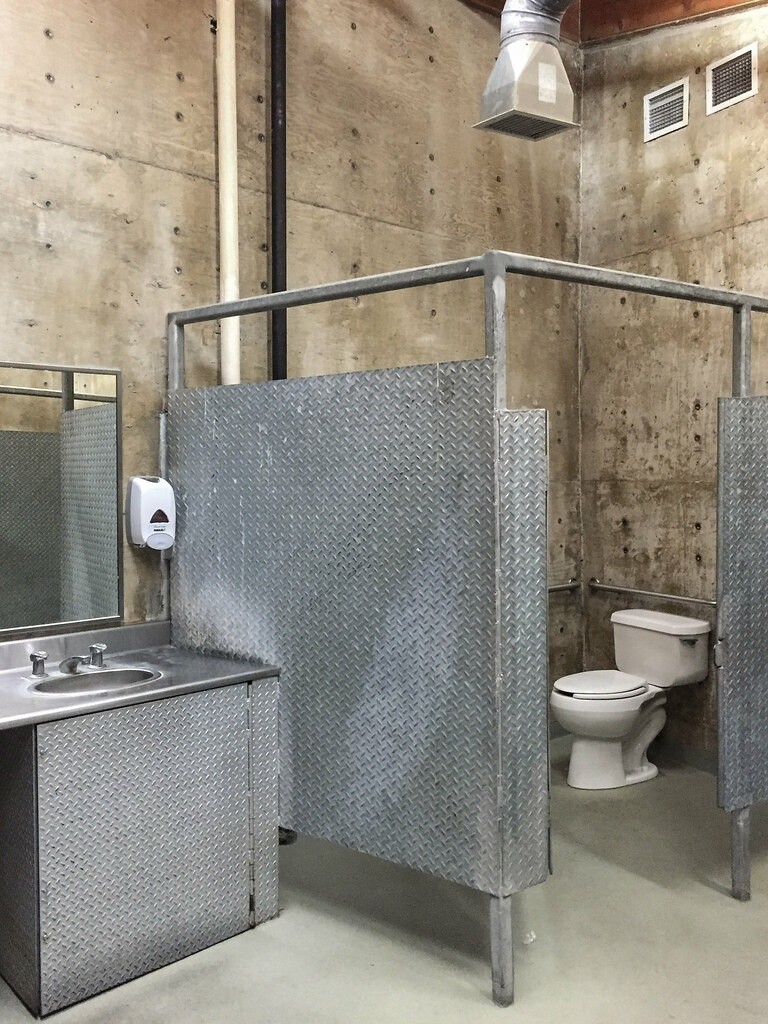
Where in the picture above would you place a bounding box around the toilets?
[548,608,711,790]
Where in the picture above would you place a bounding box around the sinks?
[28,667,162,696]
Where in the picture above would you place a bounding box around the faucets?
[58,654,92,675]
